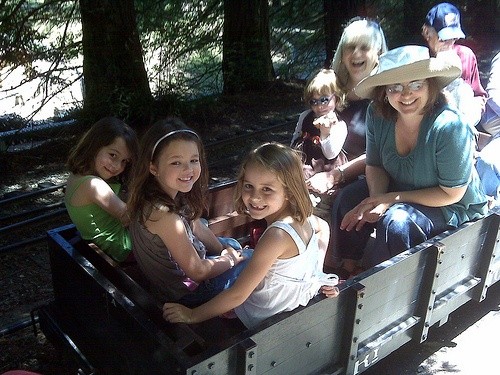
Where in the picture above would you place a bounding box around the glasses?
[387,81,424,92]
[310,93,336,106]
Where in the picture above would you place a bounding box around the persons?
[64,118,139,263]
[124,118,247,309]
[290,3,500,281]
[163,142,340,329]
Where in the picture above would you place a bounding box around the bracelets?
[337,168,345,185]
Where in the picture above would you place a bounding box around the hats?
[426,3,466,40]
[354,44,461,99]
